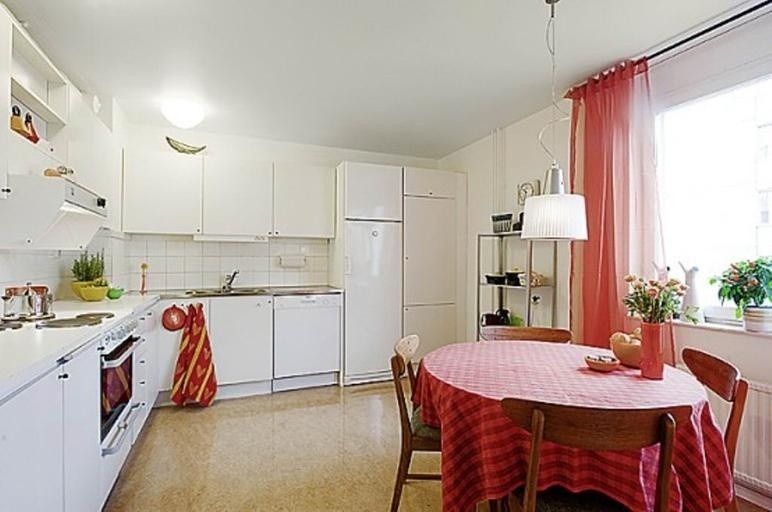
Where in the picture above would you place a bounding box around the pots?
[1,283,53,317]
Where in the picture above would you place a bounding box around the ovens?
[96,323,147,496]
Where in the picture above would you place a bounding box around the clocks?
[515,177,539,207]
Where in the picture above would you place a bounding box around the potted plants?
[73,252,108,304]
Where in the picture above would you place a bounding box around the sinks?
[191,290,267,296]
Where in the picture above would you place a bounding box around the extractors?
[1,174,107,253]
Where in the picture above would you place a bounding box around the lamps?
[160,99,206,130]
[521,0,590,240]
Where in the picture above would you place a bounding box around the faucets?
[220,269,240,290]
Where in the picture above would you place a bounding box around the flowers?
[621,271,695,325]
[713,257,772,306]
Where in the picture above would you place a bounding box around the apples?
[107,288,122,298]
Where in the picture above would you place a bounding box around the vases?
[736,309,771,332]
[636,322,668,380]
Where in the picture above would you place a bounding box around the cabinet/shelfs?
[157,300,207,404]
[404,305,457,373]
[0,353,63,512]
[1,5,11,196]
[337,160,402,221]
[12,12,70,176]
[70,82,122,231]
[122,158,203,233]
[404,197,456,303]
[404,165,455,199]
[208,297,272,398]
[205,161,335,237]
[273,294,338,392]
[477,232,557,341]
[64,336,100,512]
[136,301,156,442]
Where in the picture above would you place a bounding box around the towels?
[184,304,216,409]
[172,303,197,407]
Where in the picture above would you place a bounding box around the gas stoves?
[40,310,134,335]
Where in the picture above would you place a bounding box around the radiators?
[725,379,771,509]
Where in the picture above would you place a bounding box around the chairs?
[683,345,748,512]
[391,334,453,512]
[506,397,693,512]
[480,327,572,344]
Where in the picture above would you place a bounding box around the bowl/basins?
[609,337,642,369]
[585,354,621,372]
